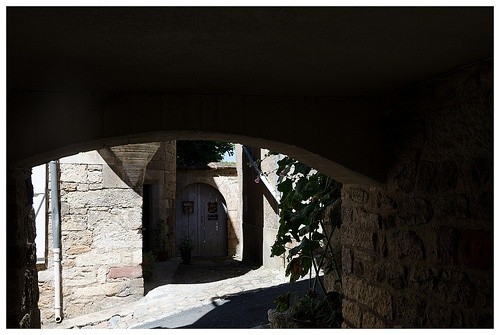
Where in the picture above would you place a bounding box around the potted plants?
[176,230,194,265]
[267,150,343,329]
[151,219,169,263]
[142,250,158,279]
